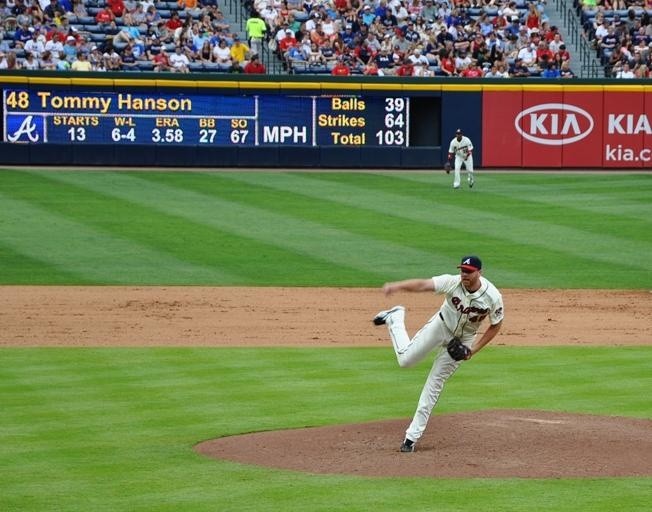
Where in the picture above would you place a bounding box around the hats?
[232,32,240,40]
[92,46,99,51]
[281,1,569,71]
[69,26,77,32]
[457,256,482,271]
[152,34,160,41]
[456,129,463,134]
[66,35,75,41]
[161,46,167,51]
[35,25,40,30]
[50,22,57,27]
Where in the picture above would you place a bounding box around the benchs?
[1,0,652,80]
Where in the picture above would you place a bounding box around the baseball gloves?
[446,338,472,361]
[445,163,450,174]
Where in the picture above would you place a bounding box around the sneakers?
[401,438,416,453]
[373,305,405,326]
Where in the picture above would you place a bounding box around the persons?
[372,255,503,452]
[447,127,474,189]
